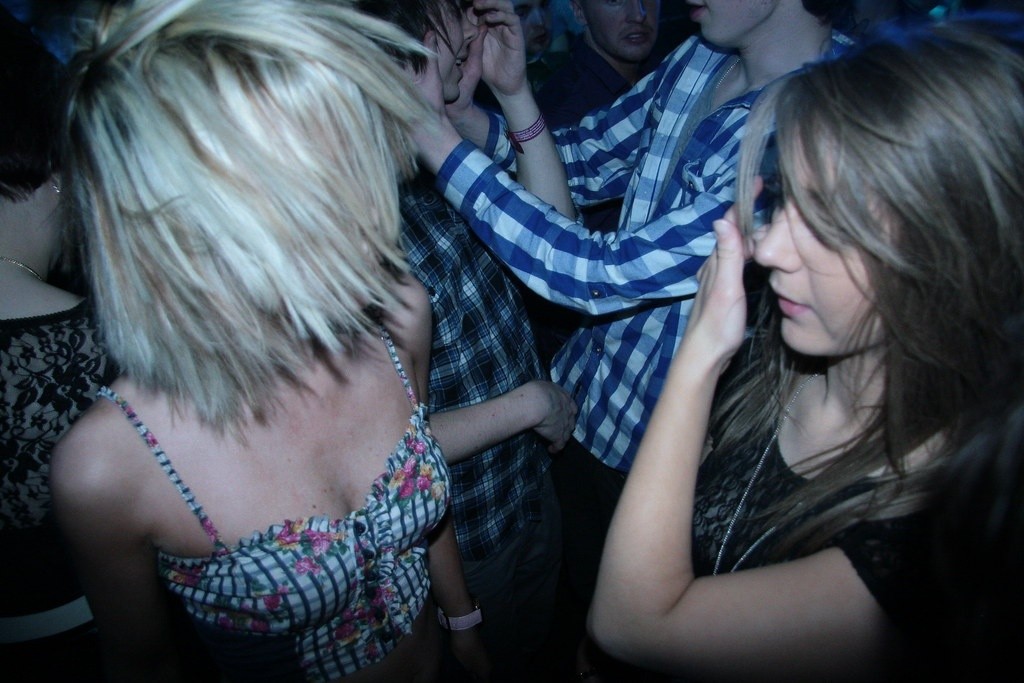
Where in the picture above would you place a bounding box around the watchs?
[438,599,483,630]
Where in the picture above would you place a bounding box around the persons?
[506,115,546,154]
[581,24,1022,683]
[400,1,853,604]
[538,0,669,359]
[354,2,579,683]
[52,0,487,683]
[512,0,556,107]
[0,29,120,683]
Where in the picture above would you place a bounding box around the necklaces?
[716,57,740,90]
[0,256,45,282]
